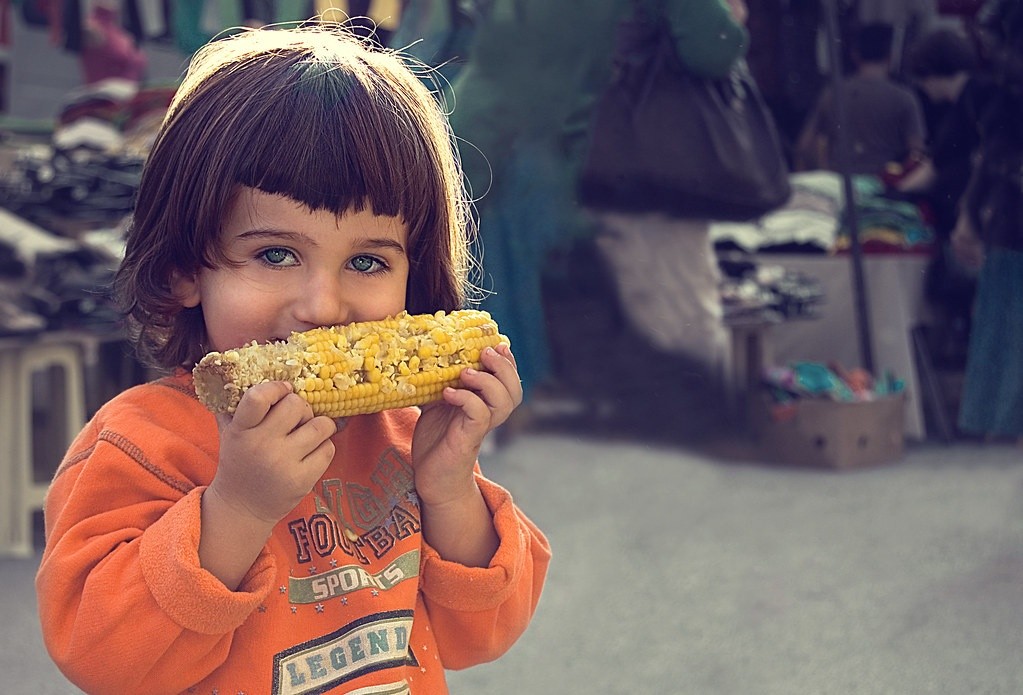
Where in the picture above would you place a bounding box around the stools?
[0,342,88,564]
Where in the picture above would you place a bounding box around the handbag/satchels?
[950,199,985,277]
[576,0,789,223]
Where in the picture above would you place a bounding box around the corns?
[192,308,511,418]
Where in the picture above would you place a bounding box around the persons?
[33,6,553,694]
[792,1,1023,444]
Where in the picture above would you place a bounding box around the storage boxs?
[758,390,912,472]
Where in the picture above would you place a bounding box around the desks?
[727,243,936,444]
